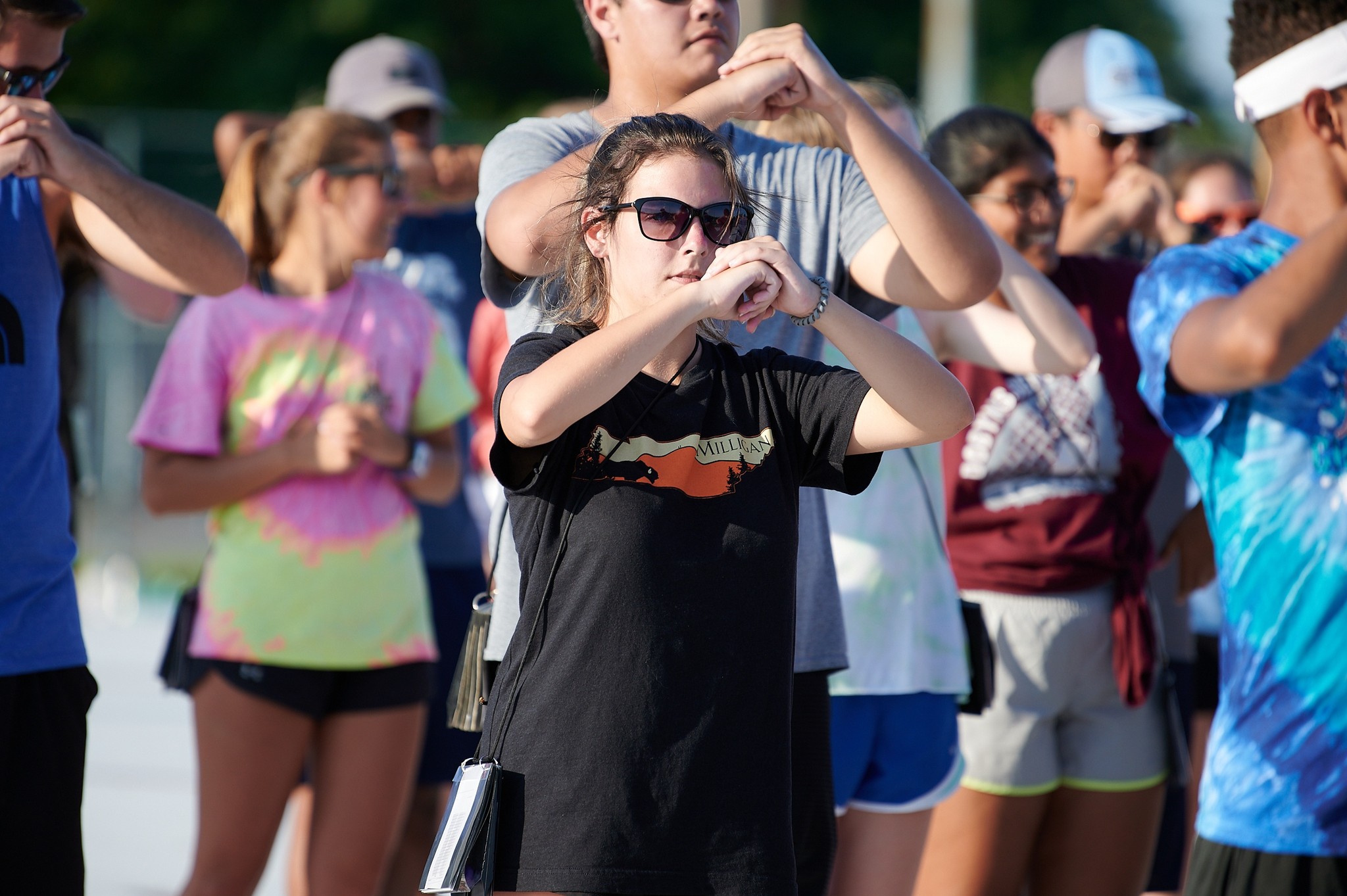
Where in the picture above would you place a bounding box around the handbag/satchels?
[157,589,202,693]
[956,597,997,717]
[445,598,495,732]
[416,759,502,896]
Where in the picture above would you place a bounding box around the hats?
[325,33,449,120]
[1029,25,1199,135]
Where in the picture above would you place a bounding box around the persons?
[492,110,972,896]
[127,102,481,896]
[471,0,1004,896]
[215,35,490,896]
[750,30,1268,896]
[1130,1,1346,896]
[0,0,247,896]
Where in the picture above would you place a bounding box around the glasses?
[599,196,755,247]
[977,176,1075,217]
[291,164,408,199]
[1098,123,1173,153]
[0,52,76,98]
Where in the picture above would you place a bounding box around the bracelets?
[788,275,829,327]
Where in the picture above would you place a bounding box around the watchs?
[392,436,433,481]
